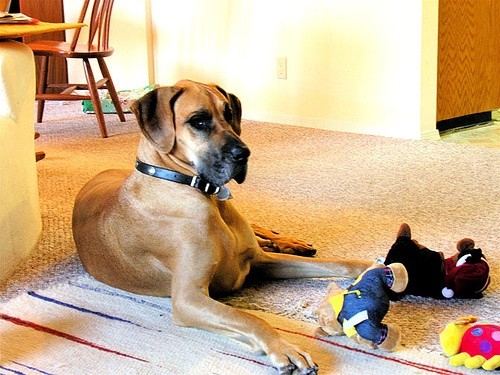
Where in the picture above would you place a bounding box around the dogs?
[71,79,383,375]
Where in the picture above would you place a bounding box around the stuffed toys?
[314,261,411,353]
[382,223,491,302]
[438,313,500,370]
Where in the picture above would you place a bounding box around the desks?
[0,21,88,40]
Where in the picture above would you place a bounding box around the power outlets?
[276,55,290,81]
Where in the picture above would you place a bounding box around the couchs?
[0,44,42,285]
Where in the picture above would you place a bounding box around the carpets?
[0,273,499,375]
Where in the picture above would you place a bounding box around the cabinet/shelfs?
[0,1,70,102]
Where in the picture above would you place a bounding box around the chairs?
[28,0,125,140]
